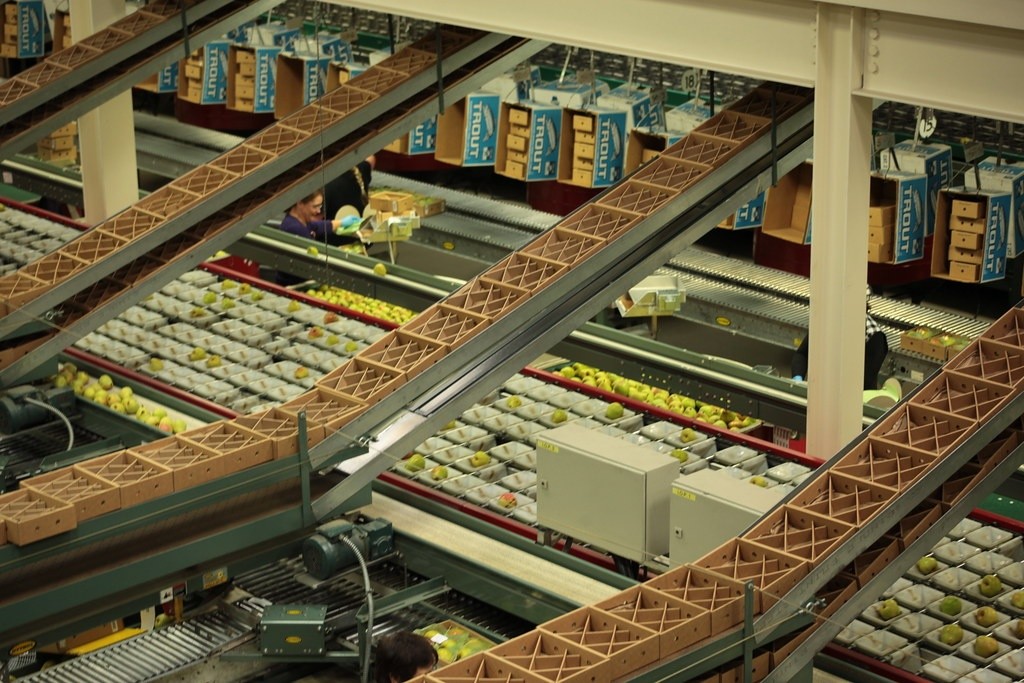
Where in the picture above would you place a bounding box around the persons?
[279,189,362,240]
[321,155,375,246]
[375,633,438,683]
[790,283,887,391]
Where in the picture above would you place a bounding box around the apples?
[941,626,963,645]
[1015,620,1024,640]
[919,558,936,573]
[981,576,1002,596]
[941,597,960,615]
[48,241,768,509]
[978,607,998,625]
[976,637,998,656]
[425,629,480,663]
[1011,592,1024,607]
[879,600,899,619]
